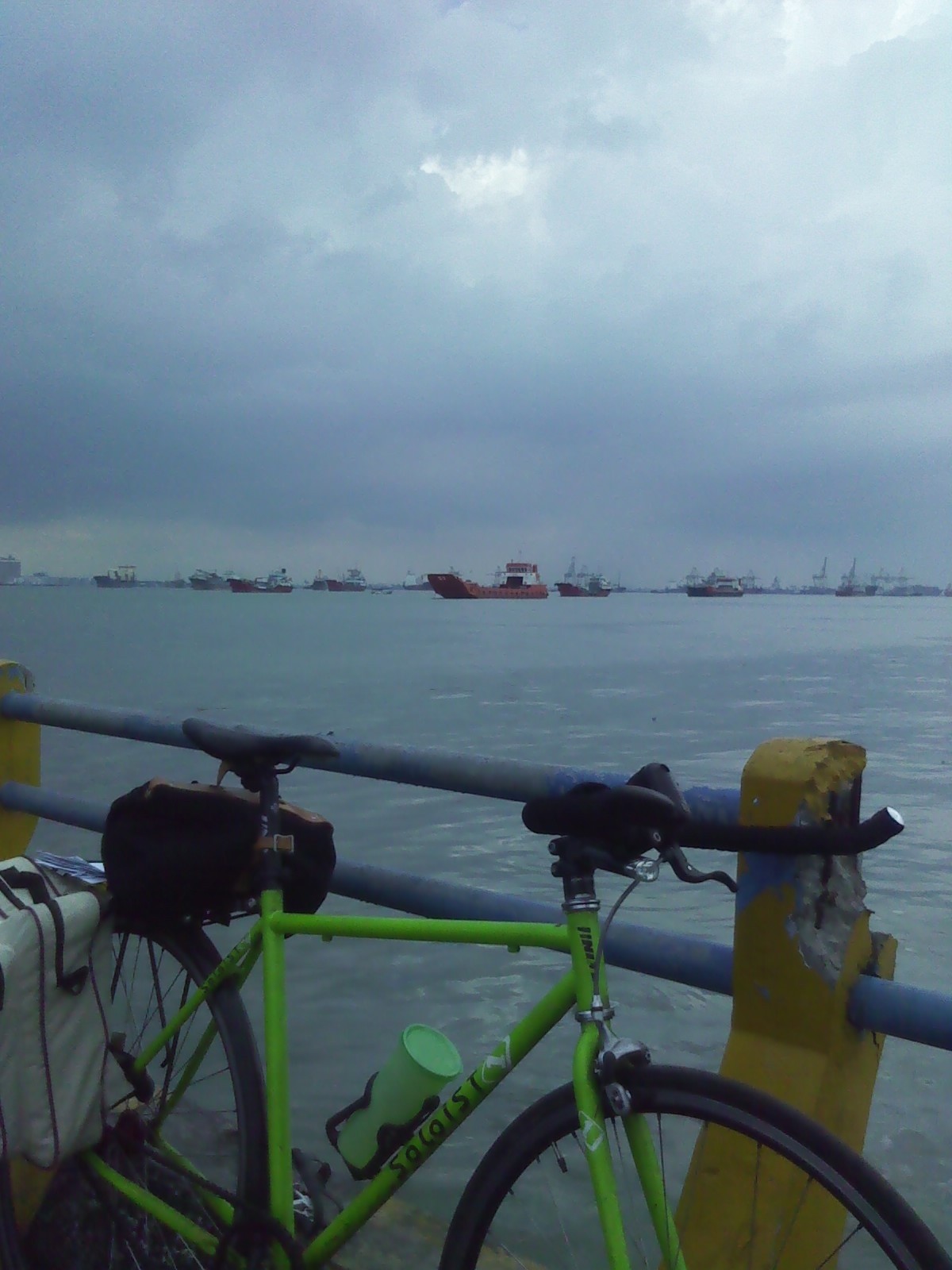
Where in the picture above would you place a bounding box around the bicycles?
[0,722,952,1270]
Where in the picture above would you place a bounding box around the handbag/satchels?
[0,853,157,1172]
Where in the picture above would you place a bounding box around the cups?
[337,1024,465,1170]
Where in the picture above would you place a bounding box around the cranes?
[812,556,829,588]
[840,557,857,586]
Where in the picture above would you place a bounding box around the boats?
[686,567,744,597]
[189,568,239,590]
[739,571,795,594]
[372,589,392,594]
[303,568,367,591]
[93,566,185,587]
[836,580,952,596]
[402,570,433,590]
[554,556,612,597]
[226,569,293,593]
[427,562,549,600]
[610,571,626,593]
[651,580,687,593]
